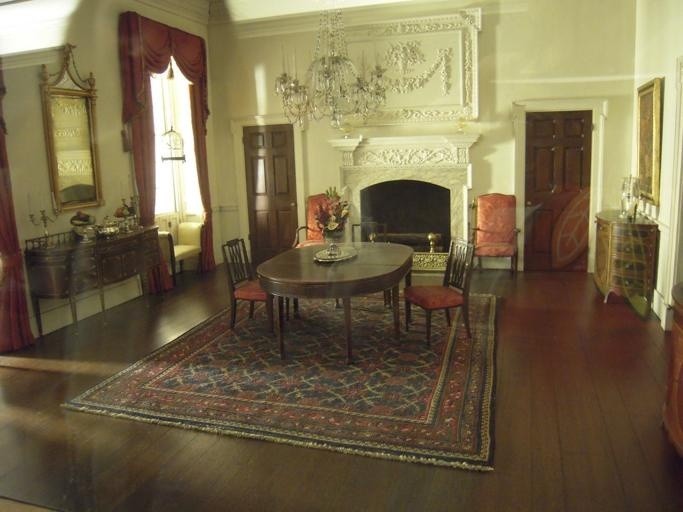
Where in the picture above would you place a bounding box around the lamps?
[275,0,388,132]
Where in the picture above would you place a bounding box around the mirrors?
[38,88,103,213]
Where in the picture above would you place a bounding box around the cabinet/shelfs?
[593,209,658,305]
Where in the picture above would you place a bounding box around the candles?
[26,190,59,215]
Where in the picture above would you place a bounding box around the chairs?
[470,194,522,277]
[156,213,204,286]
[292,193,340,249]
[220,238,280,330]
[403,238,477,347]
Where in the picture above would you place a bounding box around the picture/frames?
[635,76,662,208]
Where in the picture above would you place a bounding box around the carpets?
[58,290,500,469]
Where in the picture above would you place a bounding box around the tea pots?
[99,216,121,240]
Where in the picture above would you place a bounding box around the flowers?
[310,186,351,237]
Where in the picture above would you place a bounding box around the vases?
[324,229,343,254]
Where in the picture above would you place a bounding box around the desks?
[257,242,413,365]
[26,224,160,341]
